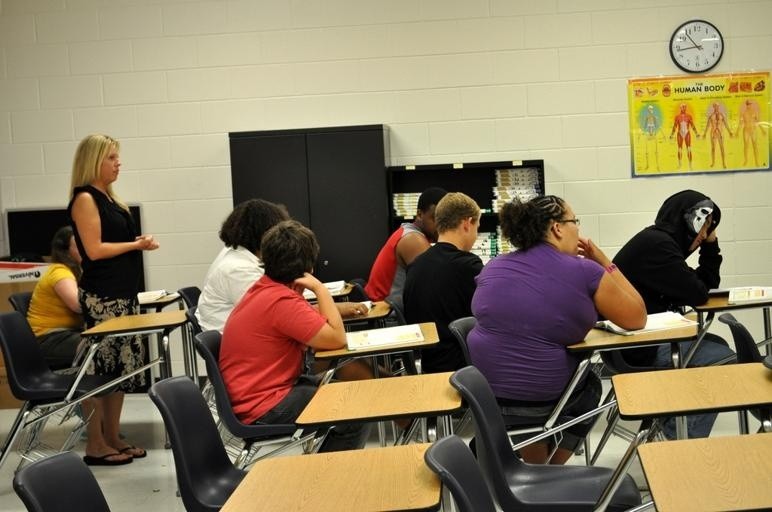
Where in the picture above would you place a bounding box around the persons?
[25,225,86,368]
[363,188,447,319]
[195,198,372,381]
[218,221,376,453]
[207,212,230,283]
[401,190,485,374]
[466,195,649,465]
[64,132,160,466]
[596,189,738,492]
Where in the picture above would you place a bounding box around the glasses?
[555,218,581,228]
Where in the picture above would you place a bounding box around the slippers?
[82,451,133,467]
[120,443,147,458]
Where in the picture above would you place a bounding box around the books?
[590,309,701,337]
[358,300,379,315]
[392,167,546,265]
[726,285,771,305]
[138,289,171,303]
[343,323,424,350]
[303,279,347,297]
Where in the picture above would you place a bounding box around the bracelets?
[605,263,618,274]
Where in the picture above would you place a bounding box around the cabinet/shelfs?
[385,159,546,268]
[226,122,393,283]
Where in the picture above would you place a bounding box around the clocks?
[668,19,725,73]
